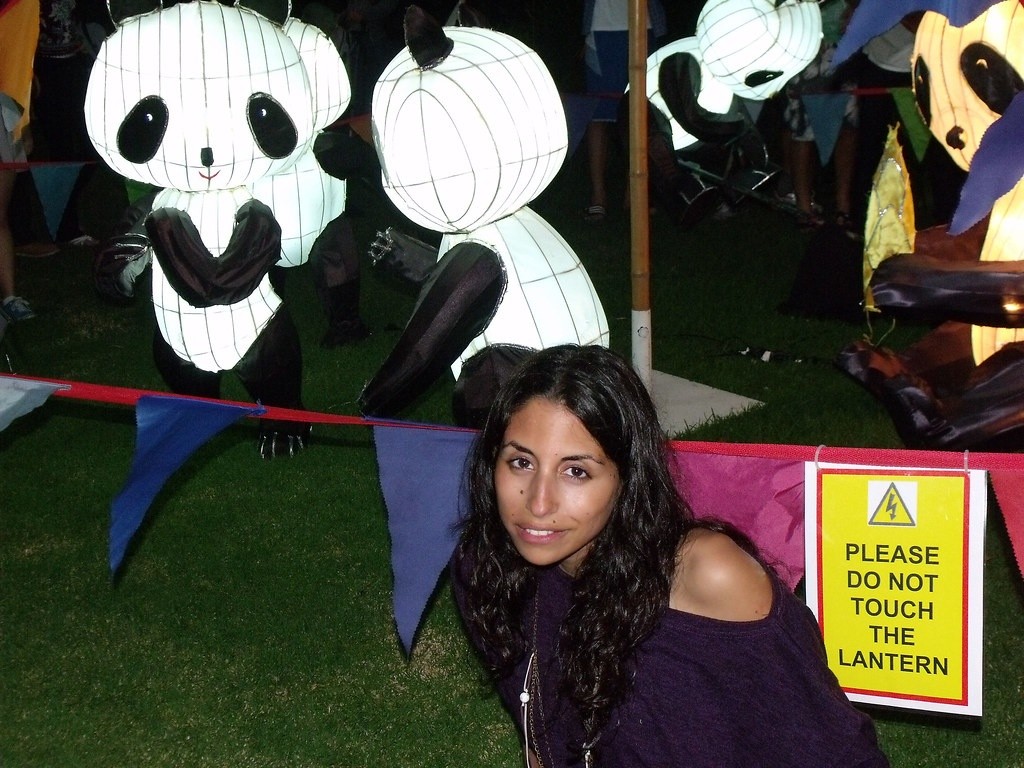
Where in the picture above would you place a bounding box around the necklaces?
[526,572,555,768]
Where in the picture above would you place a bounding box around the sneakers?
[4,296,37,324]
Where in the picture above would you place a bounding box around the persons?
[783,50,862,231]
[0,1,100,321]
[448,342,887,768]
[580,0,668,220]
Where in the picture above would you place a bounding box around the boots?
[320,275,368,349]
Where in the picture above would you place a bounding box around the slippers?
[582,204,607,227]
[621,204,661,221]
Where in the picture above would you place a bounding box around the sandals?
[69,234,98,250]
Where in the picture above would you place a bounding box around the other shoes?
[16,241,60,259]
[835,212,854,228]
[802,211,826,228]
